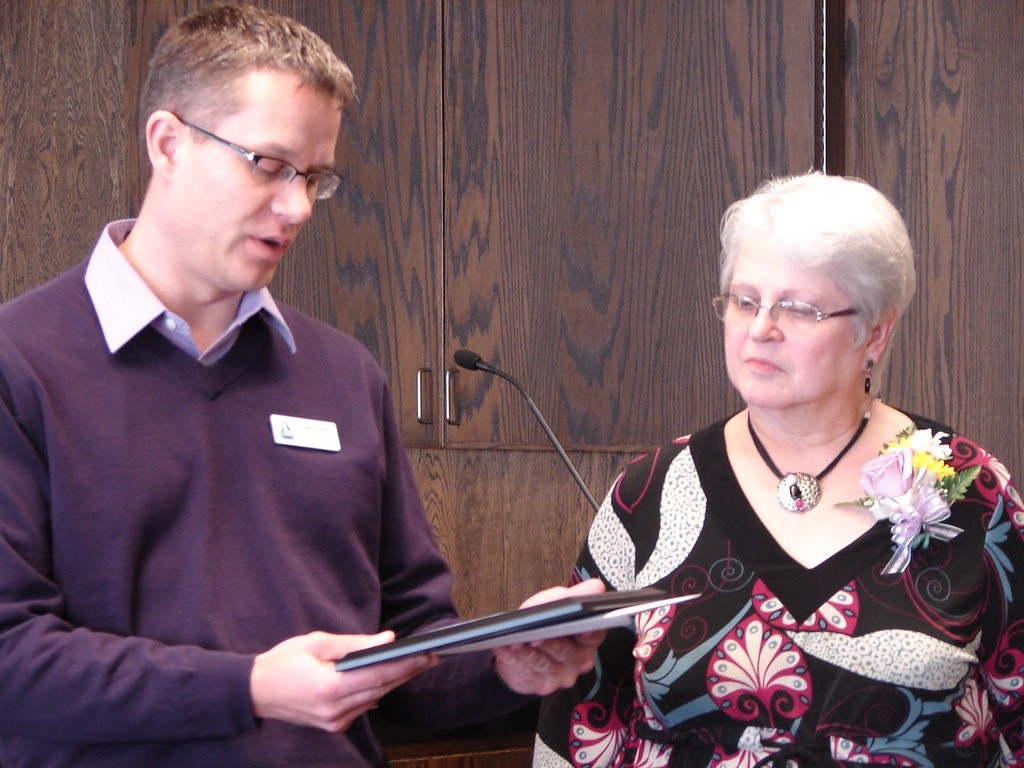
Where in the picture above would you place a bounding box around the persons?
[1,4,607,768]
[532,171,1024,768]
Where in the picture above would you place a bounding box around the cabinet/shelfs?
[126,0,846,449]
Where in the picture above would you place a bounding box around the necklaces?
[747,408,871,512]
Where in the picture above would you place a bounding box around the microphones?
[454,350,602,512]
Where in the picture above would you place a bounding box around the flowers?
[835,426,981,575]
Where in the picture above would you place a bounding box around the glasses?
[712,292,857,330]
[178,120,344,200]
